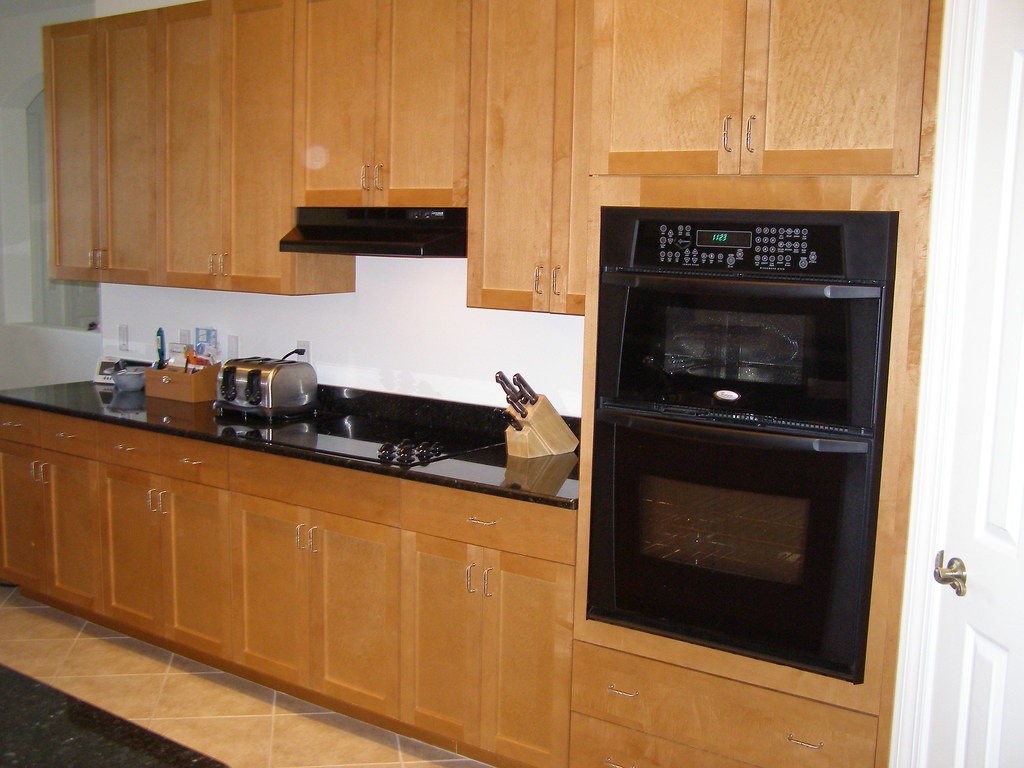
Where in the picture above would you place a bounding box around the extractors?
[277,203,472,261]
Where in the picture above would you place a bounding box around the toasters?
[216,356,320,419]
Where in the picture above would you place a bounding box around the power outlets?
[296,340,312,365]
[179,329,192,344]
[118,324,129,351]
[226,335,240,360]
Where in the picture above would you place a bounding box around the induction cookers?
[264,385,479,470]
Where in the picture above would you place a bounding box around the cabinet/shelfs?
[155,0,356,296]
[228,446,401,735]
[41,9,155,286]
[467,0,595,316]
[0,402,104,626]
[401,480,578,768]
[96,422,230,676]
[589,0,944,178]
[569,639,878,768]
[291,0,469,207]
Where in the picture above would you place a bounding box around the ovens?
[578,198,904,685]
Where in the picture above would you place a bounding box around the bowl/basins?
[111,372,144,391]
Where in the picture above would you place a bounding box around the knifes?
[492,370,540,433]
